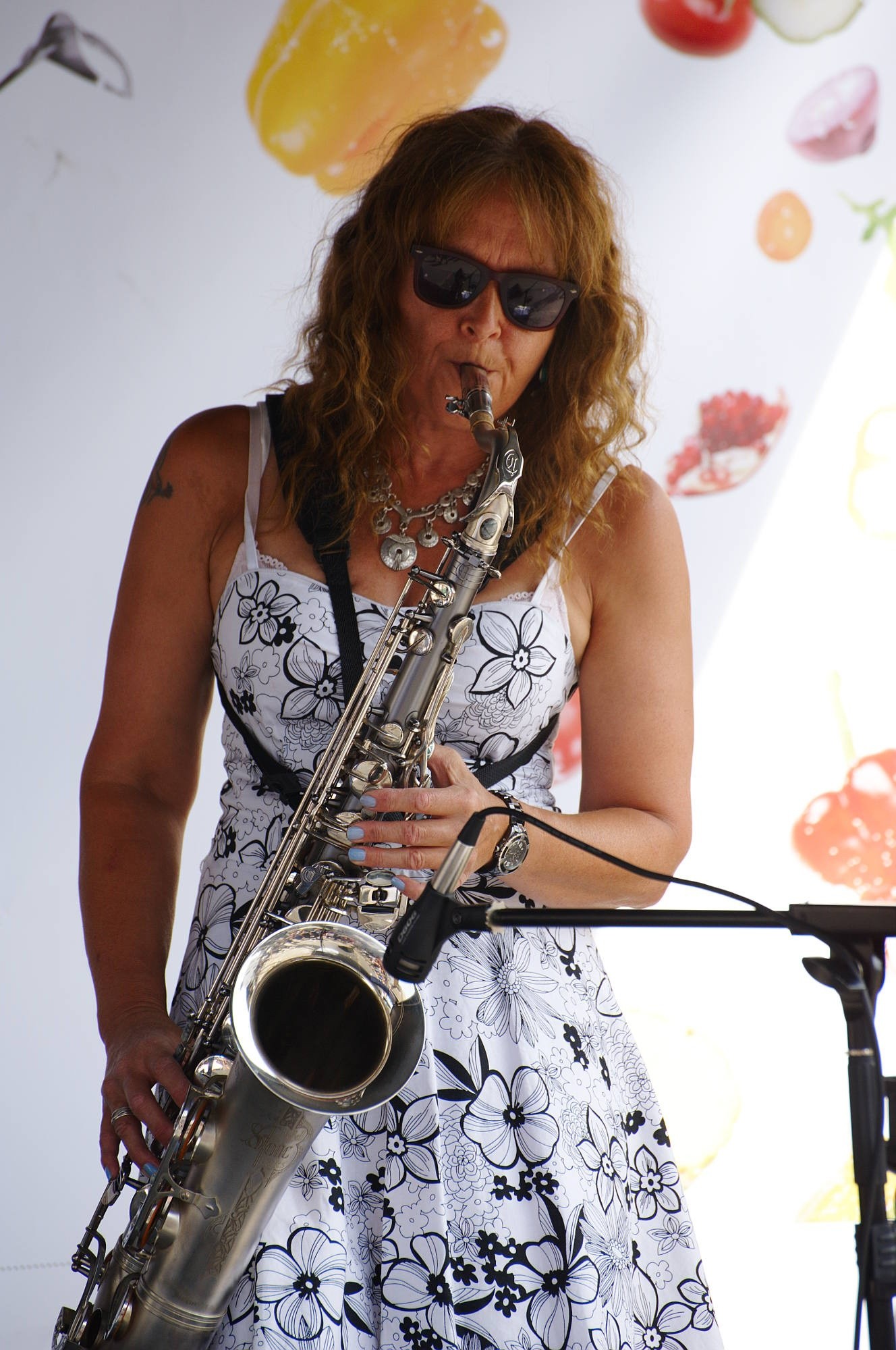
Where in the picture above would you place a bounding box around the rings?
[111,1106,133,1124]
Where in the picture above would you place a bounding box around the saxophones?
[45,360,530,1350]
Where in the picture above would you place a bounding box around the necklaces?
[357,451,490,571]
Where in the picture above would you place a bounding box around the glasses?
[409,235,578,334]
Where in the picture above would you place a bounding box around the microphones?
[383,811,488,984]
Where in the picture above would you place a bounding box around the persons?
[79,108,727,1350]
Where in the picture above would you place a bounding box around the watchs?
[473,789,530,877]
